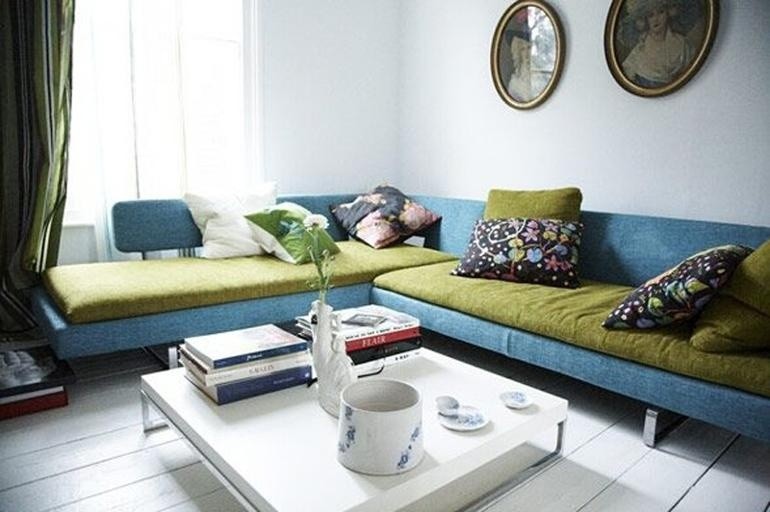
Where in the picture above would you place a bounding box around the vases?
[308,300,357,417]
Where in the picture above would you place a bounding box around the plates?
[500,390,534,410]
[440,405,488,433]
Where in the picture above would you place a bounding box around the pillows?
[689,295,770,352]
[184,183,278,259]
[601,244,753,330]
[450,218,589,290]
[245,201,340,265]
[720,239,770,315]
[484,188,582,222]
[329,183,443,249]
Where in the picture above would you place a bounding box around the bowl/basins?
[436,396,460,416]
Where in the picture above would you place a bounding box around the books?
[177,304,422,406]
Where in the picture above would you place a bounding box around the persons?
[507,36,547,103]
[621,5,694,88]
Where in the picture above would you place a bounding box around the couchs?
[29,193,769,448]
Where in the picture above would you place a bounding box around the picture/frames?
[490,0,565,110]
[603,0,718,98]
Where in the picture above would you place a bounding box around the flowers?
[300,213,339,299]
